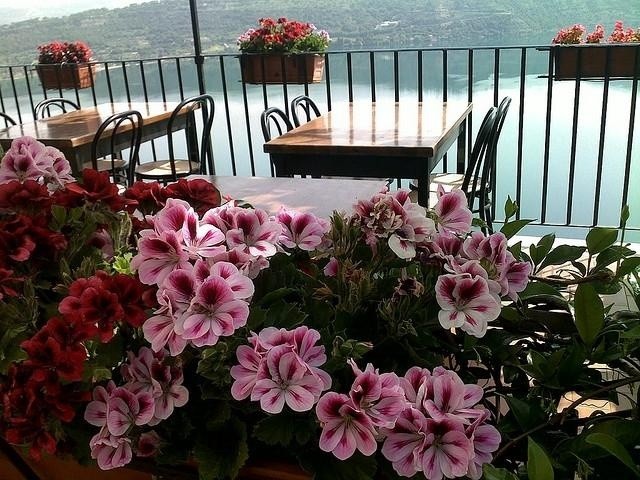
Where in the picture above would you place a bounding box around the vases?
[237,52,325,84]
[12,444,325,480]
[554,43,640,78]
[35,62,96,90]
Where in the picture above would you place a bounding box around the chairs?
[408,95,512,222]
[260,107,393,193]
[408,107,498,238]
[291,96,321,127]
[82,110,143,197]
[0,113,16,129]
[33,97,129,186]
[134,94,215,188]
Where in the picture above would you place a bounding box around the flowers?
[35,40,93,63]
[551,19,640,44]
[237,17,333,53]
[0,136,640,480]
